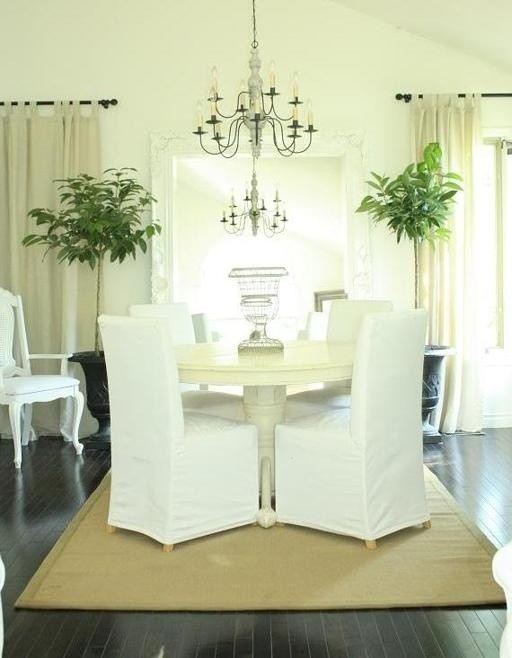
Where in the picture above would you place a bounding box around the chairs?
[128,302,244,421]
[287,301,394,409]
[97,314,260,551]
[0,288,86,467]
[273,307,430,548]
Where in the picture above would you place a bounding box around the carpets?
[13,458,508,613]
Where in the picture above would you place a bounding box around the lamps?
[221,153,288,238]
[194,1,320,159]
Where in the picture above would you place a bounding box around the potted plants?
[355,141,465,444]
[18,166,162,439]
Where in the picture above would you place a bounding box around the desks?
[175,341,355,531]
